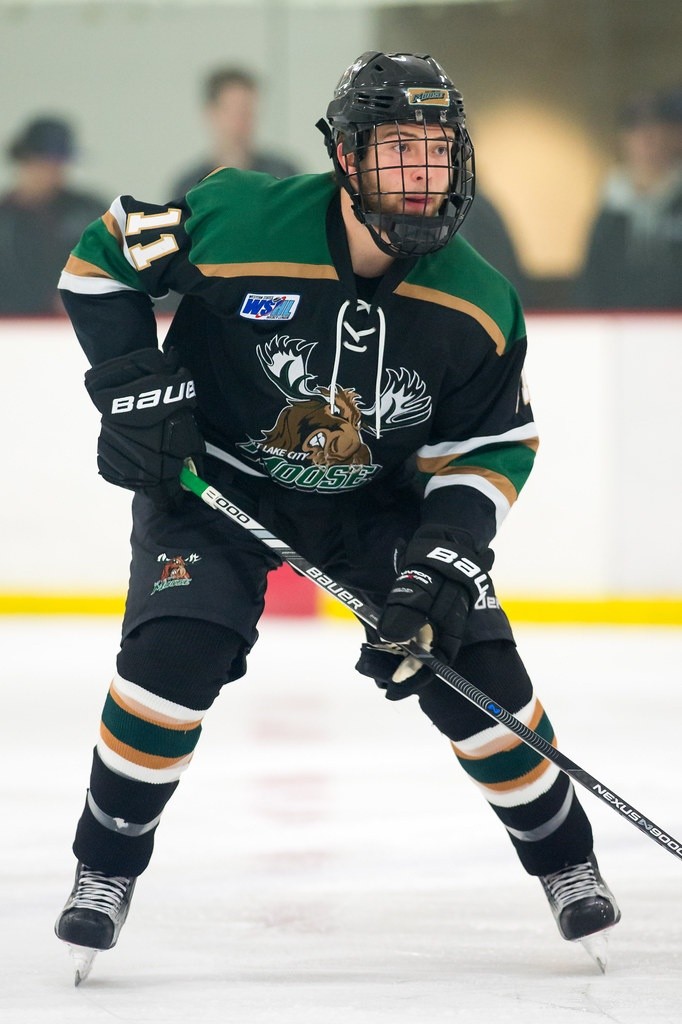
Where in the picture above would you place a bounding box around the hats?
[10,118,74,162]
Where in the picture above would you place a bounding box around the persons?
[581,96,681,312]
[151,66,311,312]
[0,115,115,318]
[50,52,620,986]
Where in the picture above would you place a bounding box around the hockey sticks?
[177,465,682,863]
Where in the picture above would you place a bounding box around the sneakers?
[538,849,622,972]
[54,863,137,987]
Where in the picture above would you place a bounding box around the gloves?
[82,345,220,516]
[355,523,496,700]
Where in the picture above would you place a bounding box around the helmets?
[326,49,467,152]
